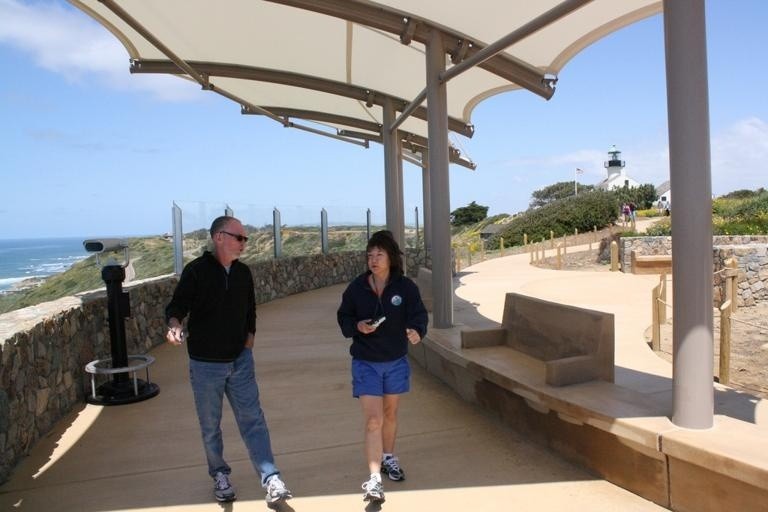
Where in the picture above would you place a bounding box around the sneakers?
[210,472,237,503]
[263,476,294,503]
[362,474,384,502]
[382,455,406,481]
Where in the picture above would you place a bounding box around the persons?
[335,227,430,504]
[628,200,637,230]
[657,201,664,217]
[620,201,630,229]
[663,201,671,217]
[162,216,296,505]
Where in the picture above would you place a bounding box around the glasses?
[221,231,248,243]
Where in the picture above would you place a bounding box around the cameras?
[369,311,391,327]
